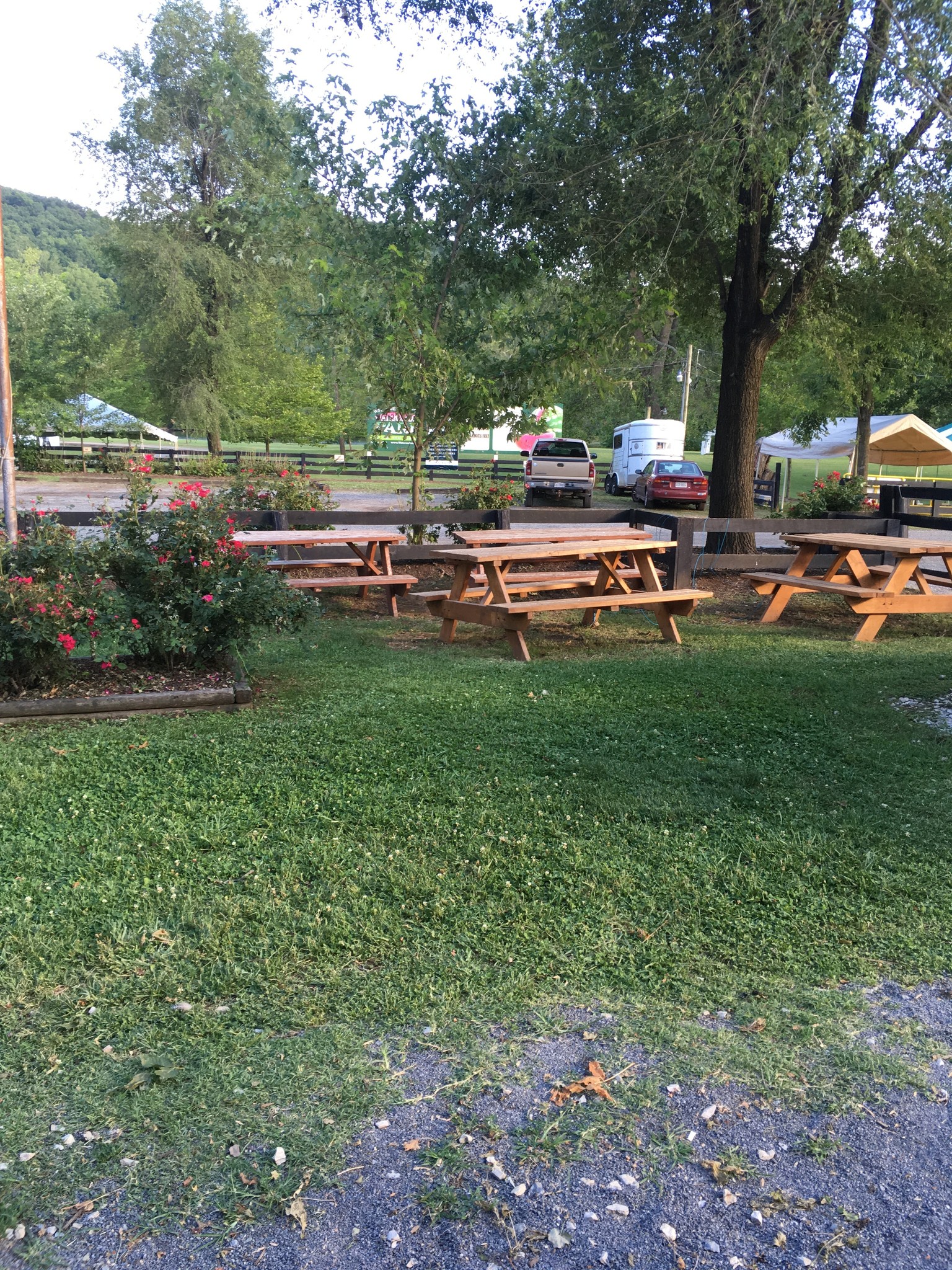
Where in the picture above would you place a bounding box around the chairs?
[570,449,581,455]
[659,464,669,473]
[673,467,692,474]
[539,447,550,455]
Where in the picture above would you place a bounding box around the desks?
[450,527,653,591]
[227,530,407,618]
[429,538,681,662]
[756,532,952,641]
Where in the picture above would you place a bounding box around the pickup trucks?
[520,437,598,508]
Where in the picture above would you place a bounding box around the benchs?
[408,553,713,632]
[284,574,418,599]
[740,565,952,614]
[258,559,380,570]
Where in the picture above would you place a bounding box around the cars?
[631,459,708,511]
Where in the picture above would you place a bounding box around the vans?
[604,418,686,497]
